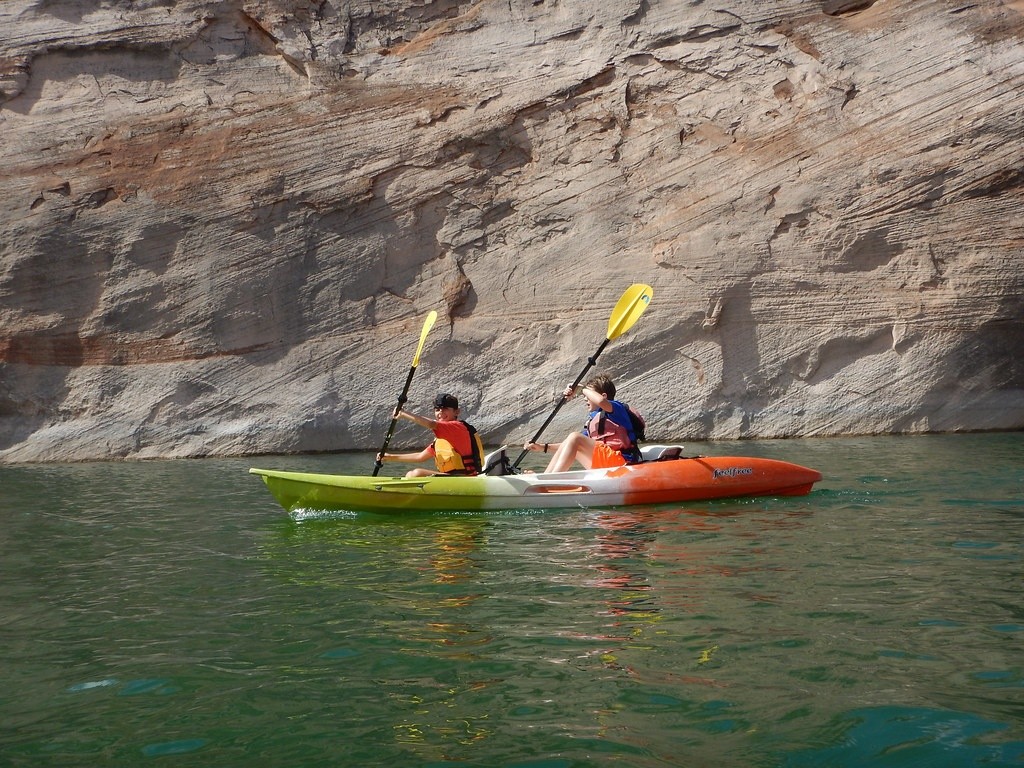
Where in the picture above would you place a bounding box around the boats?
[247,455,820,512]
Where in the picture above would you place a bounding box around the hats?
[431,393,458,409]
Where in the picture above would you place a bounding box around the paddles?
[507,283,654,474]
[372,310,437,477]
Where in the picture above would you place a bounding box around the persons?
[523,374,645,475]
[375,393,485,478]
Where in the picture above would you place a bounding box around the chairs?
[640,443,684,460]
[481,444,509,476]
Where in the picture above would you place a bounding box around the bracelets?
[544,443,549,453]
